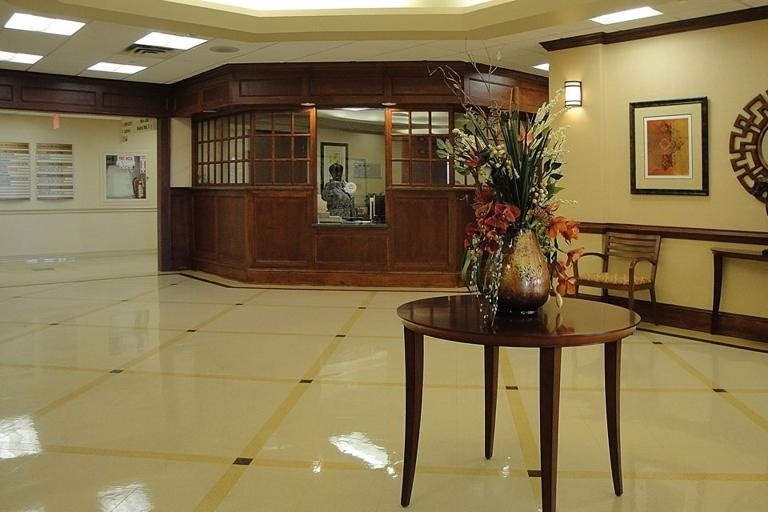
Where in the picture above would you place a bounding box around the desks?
[394,295,643,512]
[710,246,767,333]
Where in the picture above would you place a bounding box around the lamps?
[563,80,581,106]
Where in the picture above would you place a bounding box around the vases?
[483,228,552,316]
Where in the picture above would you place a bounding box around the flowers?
[423,42,581,328]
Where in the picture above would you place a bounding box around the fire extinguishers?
[133,174,145,198]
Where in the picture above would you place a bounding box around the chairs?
[573,232,662,334]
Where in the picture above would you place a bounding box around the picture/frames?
[628,96,708,196]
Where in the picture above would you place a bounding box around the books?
[319,212,330,218]
[319,221,342,224]
[319,216,340,222]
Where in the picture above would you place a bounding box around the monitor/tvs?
[367,195,384,215]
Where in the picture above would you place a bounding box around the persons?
[321,161,357,220]
